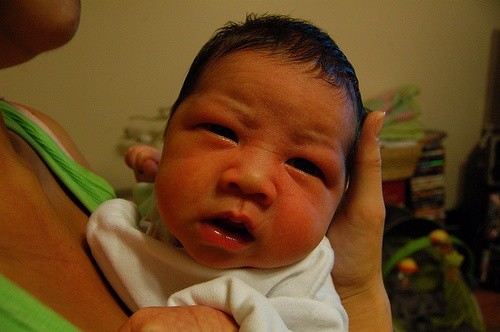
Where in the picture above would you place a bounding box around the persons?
[0,0,393,332]
[85,11,375,332]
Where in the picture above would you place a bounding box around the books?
[409,131,448,221]
